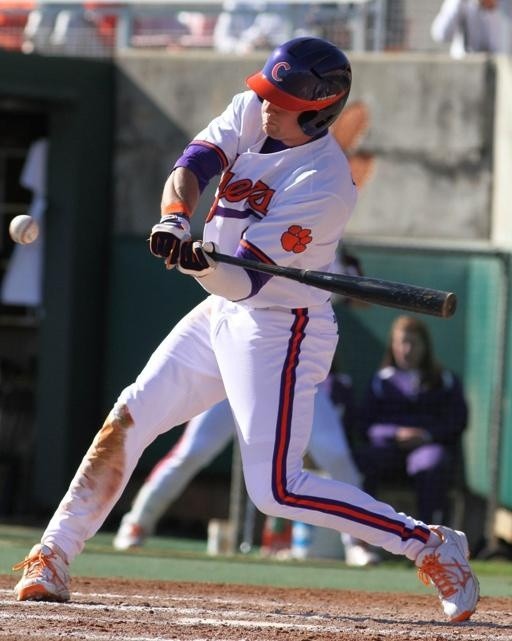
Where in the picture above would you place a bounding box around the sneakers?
[415,525,480,622]
[113,523,143,549]
[346,546,378,567]
[14,544,70,601]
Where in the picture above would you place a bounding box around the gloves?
[177,233,220,277]
[150,215,190,270]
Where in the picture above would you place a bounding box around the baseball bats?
[204,251,457,317]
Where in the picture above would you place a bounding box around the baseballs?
[10,215,39,244]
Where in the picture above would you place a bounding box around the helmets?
[247,37,352,136]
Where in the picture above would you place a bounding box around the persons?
[358,315,469,571]
[104,248,382,569]
[16,35,481,622]
[429,0,512,56]
[327,360,379,501]
